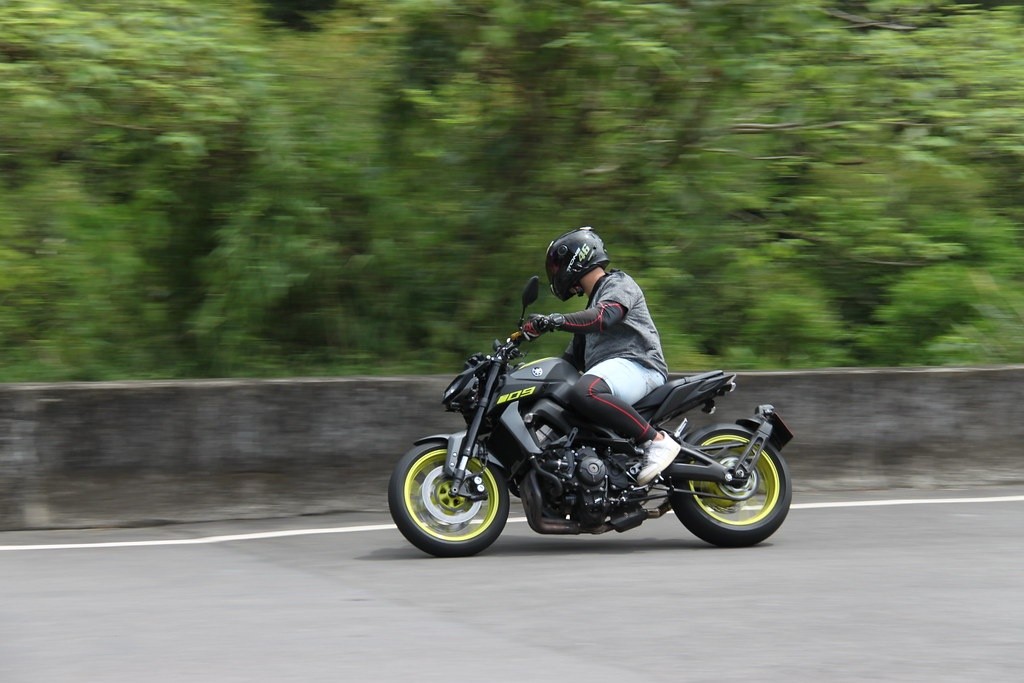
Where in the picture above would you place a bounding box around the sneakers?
[636,430,681,486]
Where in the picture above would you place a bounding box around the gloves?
[520,312,565,342]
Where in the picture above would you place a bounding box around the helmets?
[545,226,609,301]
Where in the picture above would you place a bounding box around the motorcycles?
[388,274,793,559]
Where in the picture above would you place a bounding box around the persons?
[523,227,681,486]
